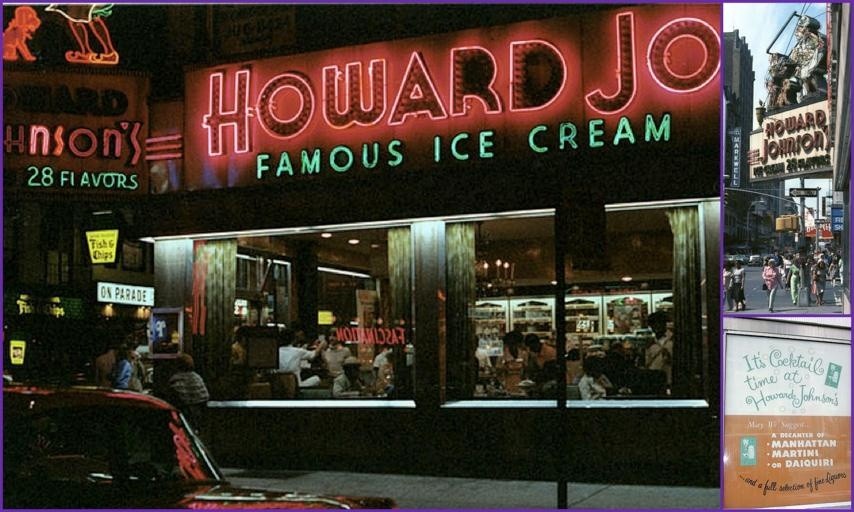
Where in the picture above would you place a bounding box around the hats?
[146,335,183,360]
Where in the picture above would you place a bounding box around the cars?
[1,377,395,508]
[232,323,288,364]
[724,249,776,268]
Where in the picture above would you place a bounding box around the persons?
[91,326,212,436]
[474,310,674,399]
[229,320,397,399]
[721,248,844,314]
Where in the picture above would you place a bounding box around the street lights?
[789,188,818,197]
[744,200,765,243]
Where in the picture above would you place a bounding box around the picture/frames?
[146,306,183,359]
